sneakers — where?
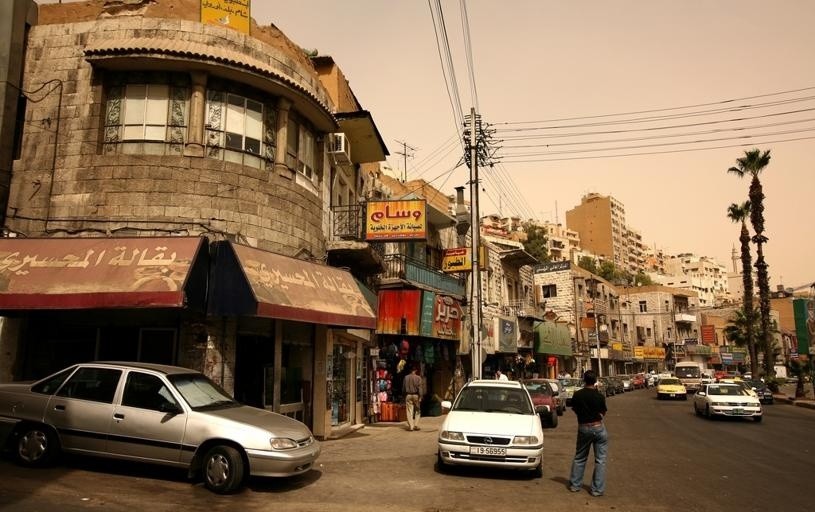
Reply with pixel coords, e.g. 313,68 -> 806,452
571,481 -> 583,491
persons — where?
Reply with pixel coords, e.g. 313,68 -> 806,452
495,370 -> 508,380
569,370 -> 608,496
558,369 -> 572,378
403,366 -> 423,431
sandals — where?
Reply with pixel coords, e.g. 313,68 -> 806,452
413,426 -> 421,430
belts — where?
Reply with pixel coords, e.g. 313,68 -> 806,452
580,421 -> 602,426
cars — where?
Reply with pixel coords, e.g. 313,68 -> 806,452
746,379 -> 775,405
0,360 -> 325,497
565,371 -> 670,403
433,377 -> 550,479
718,376 -> 759,398
692,382 -> 764,422
501,378 -> 559,429
700,369 -> 753,384
533,377 -> 568,417
657,376 -> 687,401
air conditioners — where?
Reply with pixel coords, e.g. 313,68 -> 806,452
327,131 -> 353,167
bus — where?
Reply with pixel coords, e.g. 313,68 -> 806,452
674,361 -> 702,390
